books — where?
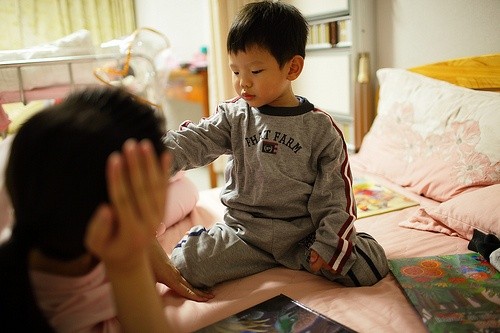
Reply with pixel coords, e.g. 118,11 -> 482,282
385,252 -> 500,333
191,291 -> 361,332
352,181 -> 419,219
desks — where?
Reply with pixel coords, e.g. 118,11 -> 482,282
167,67 -> 217,189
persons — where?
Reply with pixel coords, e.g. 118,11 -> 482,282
0,84 -> 220,332
158,0 -> 389,288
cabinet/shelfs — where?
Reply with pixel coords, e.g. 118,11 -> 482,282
291,0 -> 376,153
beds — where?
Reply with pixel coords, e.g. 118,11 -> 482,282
156,54 -> 500,332
0,53 -> 154,131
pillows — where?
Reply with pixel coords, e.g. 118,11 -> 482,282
352,69 -> 500,204
398,184 -> 500,241
